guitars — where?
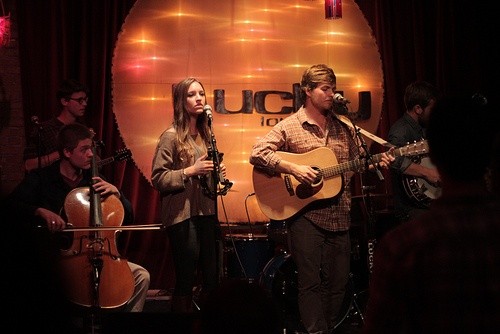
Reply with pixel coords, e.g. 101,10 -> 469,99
252,135 -> 427,220
403,136 -> 444,201
96,146 -> 132,170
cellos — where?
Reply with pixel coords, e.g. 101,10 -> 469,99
62,129 -> 136,309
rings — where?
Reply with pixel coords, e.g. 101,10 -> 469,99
51,220 -> 56,224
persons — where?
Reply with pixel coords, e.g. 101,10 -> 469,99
151,78 -> 227,334
361,84 -> 500,334
389,81 -> 444,222
21,122 -> 150,334
251,65 -> 395,334
24,83 -> 96,186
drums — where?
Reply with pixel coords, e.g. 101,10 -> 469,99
219,222 -> 239,241
223,231 -> 269,279
265,222 -> 287,256
258,251 -> 354,331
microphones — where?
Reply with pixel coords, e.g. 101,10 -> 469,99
31,116 -> 43,131
204,105 -> 213,118
333,93 -> 347,104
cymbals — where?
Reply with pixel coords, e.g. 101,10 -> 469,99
375,208 -> 396,215
200,150 -> 233,197
349,192 -> 390,200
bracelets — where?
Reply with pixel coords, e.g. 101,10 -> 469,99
182,168 -> 190,182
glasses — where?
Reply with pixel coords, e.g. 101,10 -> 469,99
69,96 -> 88,104
